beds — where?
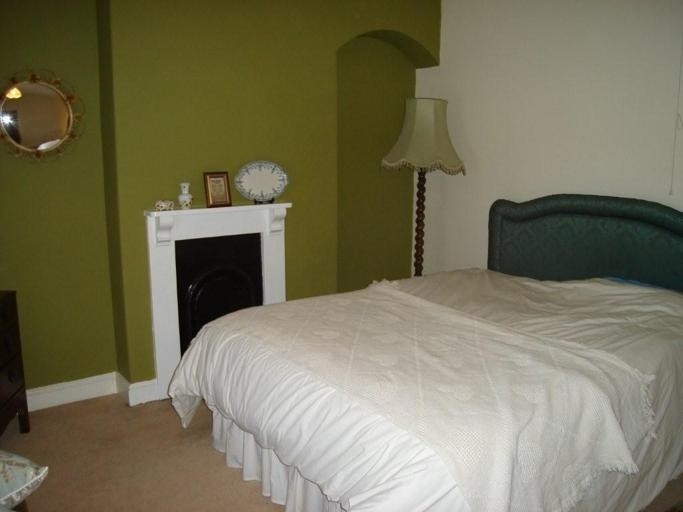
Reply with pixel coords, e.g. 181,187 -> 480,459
167,193 -> 683,512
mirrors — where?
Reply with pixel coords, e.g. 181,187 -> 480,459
0,68 -> 86,164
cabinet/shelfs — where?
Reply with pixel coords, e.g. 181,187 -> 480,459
0,290 -> 30,437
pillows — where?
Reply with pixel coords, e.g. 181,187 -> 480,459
0,449 -> 49,512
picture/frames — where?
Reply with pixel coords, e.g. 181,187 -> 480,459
203,171 -> 232,208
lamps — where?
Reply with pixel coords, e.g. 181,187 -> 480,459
379,98 -> 467,277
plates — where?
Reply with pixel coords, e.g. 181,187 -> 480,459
232,161 -> 288,201
